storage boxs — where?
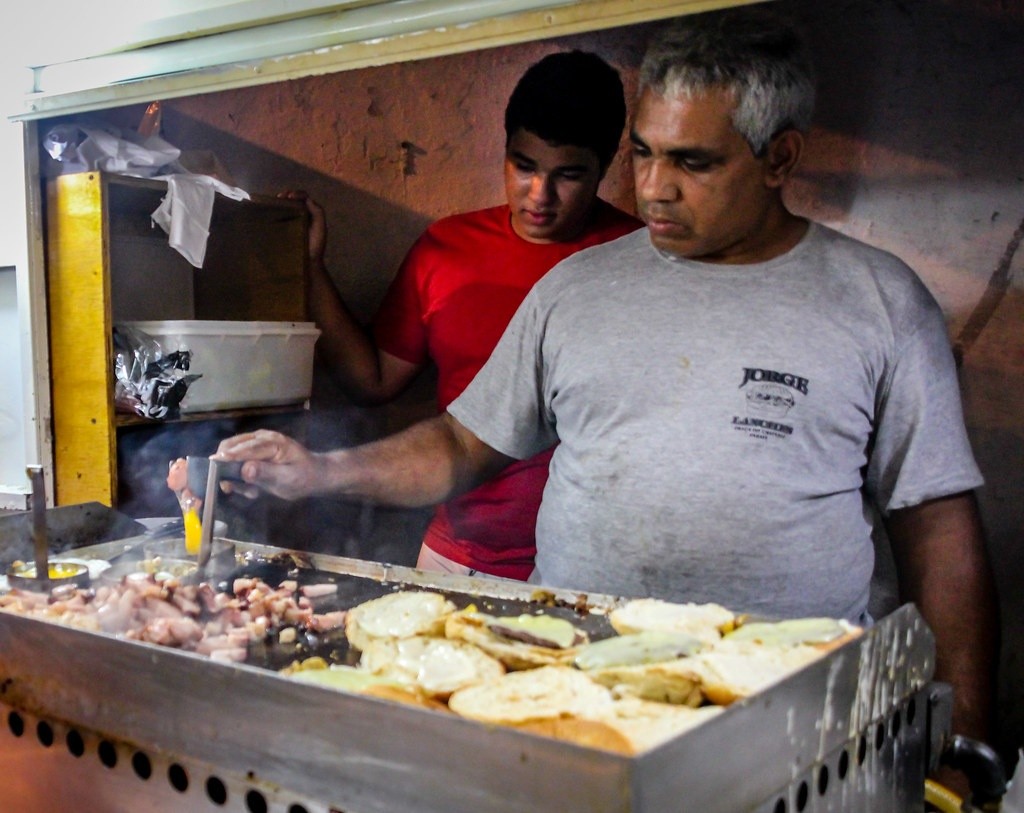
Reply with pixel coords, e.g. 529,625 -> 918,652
119,320 -> 321,414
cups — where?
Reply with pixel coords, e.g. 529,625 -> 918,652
146,537 -> 237,594
99,558 -> 201,590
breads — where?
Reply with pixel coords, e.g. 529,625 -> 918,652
279,590 -> 862,757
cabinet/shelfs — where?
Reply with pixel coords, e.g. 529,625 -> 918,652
44,170 -> 313,511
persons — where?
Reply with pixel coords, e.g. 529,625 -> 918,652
208,32 -> 986,813
277,48 -> 642,587
